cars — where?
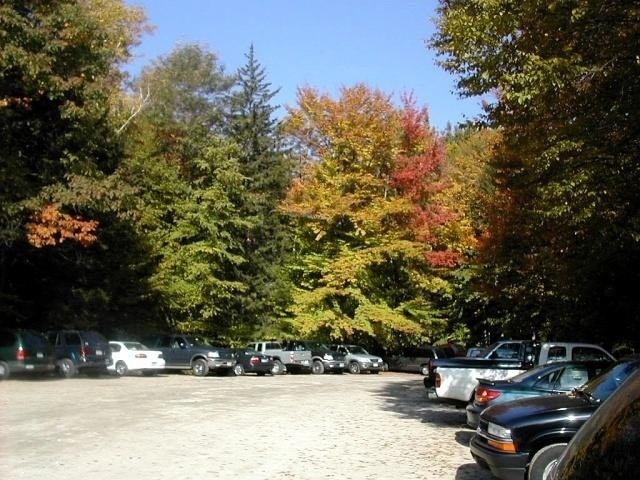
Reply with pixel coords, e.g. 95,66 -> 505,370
216,348 -> 273,376
424,339 -> 640,480
105,340 -> 168,377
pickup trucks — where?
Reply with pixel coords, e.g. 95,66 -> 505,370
247,341 -> 313,374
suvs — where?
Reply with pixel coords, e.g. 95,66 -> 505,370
146,335 -> 238,376
41,330 -> 112,377
1,329 -> 56,380
285,339 -> 344,374
330,344 -> 383,374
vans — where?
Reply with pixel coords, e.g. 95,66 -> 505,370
382,347 -> 444,376
